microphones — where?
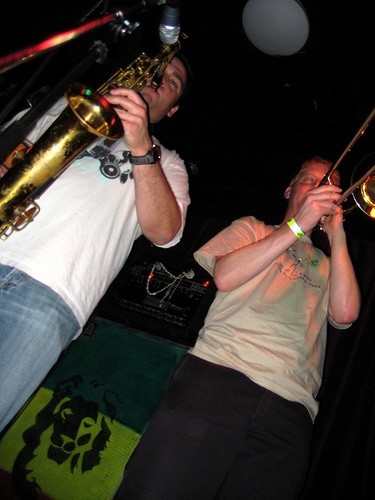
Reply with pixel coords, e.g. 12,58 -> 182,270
159,0 -> 183,44
181,260 -> 195,280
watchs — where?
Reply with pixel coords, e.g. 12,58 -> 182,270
128,145 -> 161,164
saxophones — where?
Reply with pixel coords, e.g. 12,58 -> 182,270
0,31 -> 191,241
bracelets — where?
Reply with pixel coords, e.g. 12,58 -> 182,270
286,218 -> 305,239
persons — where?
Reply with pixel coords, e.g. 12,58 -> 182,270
0,50 -> 190,432
118,158 -> 361,500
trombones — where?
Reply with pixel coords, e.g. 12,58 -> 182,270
316,108 -> 375,219
